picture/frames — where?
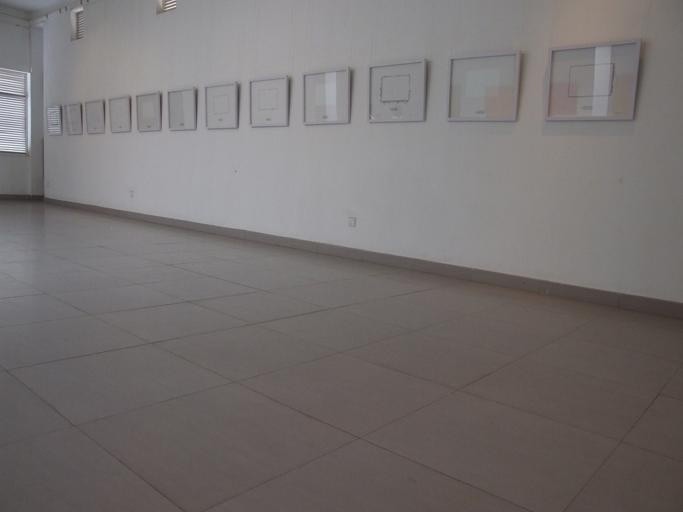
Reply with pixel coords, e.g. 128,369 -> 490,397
134,76 -> 293,133
301,58 -> 431,126
44,95 -> 131,138
445,38 -> 641,124
